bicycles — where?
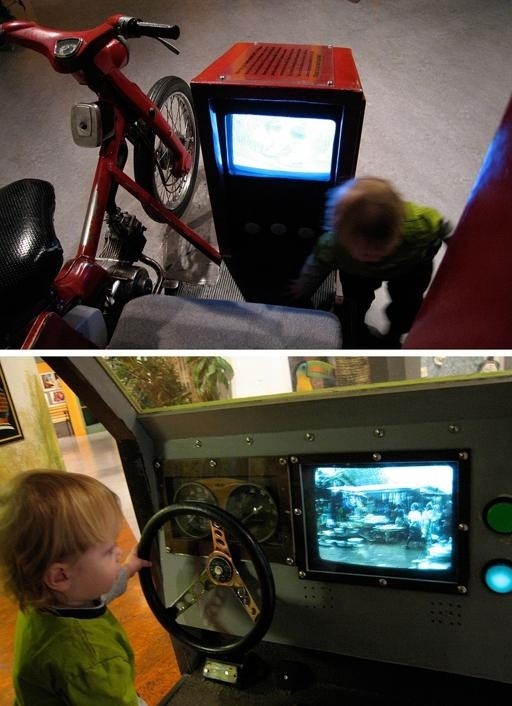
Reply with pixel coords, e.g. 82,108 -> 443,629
1,0 -> 221,349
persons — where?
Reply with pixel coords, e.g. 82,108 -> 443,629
297,174 -> 454,350
0,468 -> 157,706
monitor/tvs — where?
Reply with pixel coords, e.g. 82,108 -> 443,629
302,454 -> 464,588
218,103 -> 338,179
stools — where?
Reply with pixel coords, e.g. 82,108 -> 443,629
20,297 -> 345,351
